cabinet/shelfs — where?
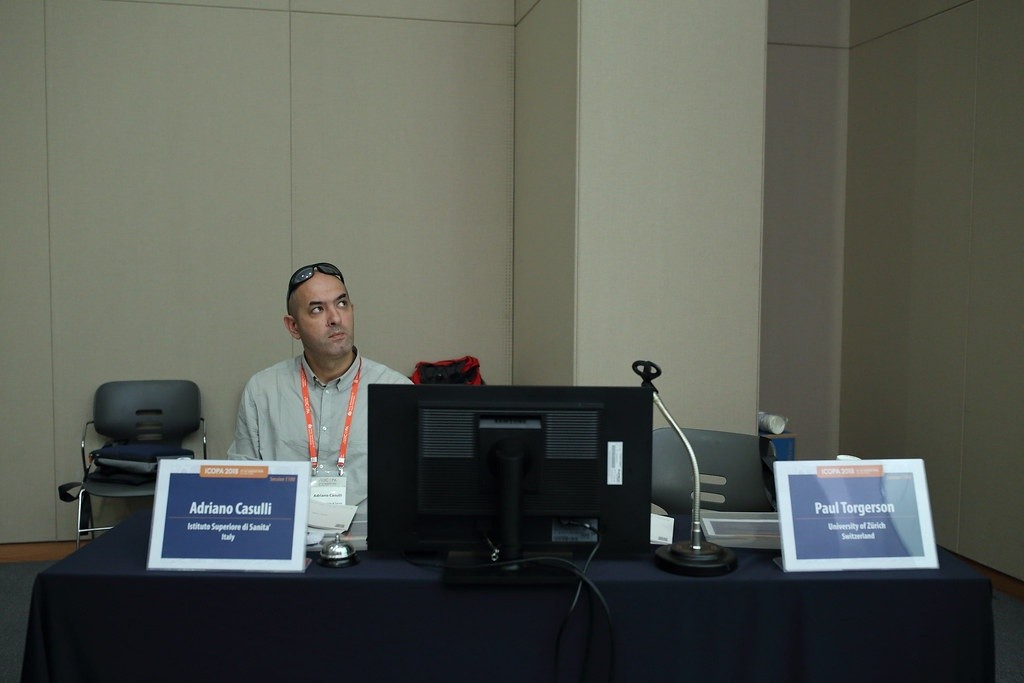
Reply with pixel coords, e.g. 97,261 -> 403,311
760,431 -> 797,499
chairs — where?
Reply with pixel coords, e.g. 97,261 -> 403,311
649,424 -> 768,519
71,378 -> 209,548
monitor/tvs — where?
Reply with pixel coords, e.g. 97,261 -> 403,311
367,383 -> 653,584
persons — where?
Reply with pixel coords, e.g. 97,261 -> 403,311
226,263 -> 415,535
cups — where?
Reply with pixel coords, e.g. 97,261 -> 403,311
836,455 -> 861,459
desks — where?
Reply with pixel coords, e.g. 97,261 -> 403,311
32,509 -> 994,683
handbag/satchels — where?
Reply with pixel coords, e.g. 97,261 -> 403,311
57,443 -> 194,537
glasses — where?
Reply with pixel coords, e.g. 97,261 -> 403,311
287,263 -> 343,297
